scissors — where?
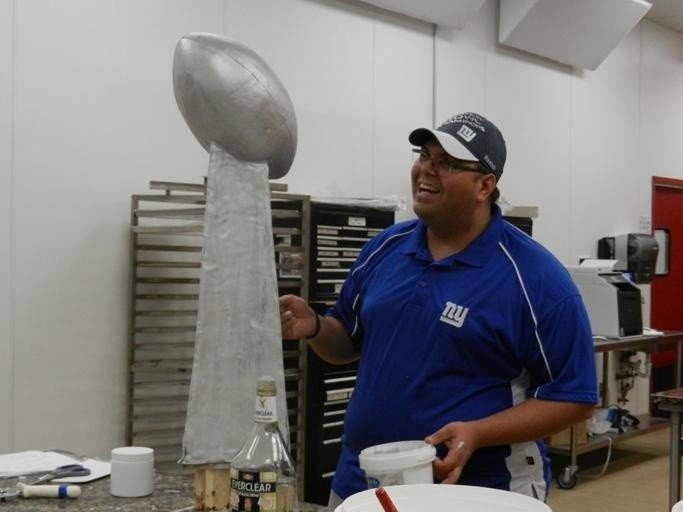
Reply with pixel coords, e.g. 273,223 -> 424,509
31,464 -> 90,485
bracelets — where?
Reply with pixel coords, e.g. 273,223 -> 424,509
308,314 -> 322,340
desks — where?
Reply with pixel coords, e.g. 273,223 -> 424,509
0,459 -> 334,512
651,384 -> 682,510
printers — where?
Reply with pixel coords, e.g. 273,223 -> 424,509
566,267 -> 644,339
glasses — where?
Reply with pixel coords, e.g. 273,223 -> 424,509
411,148 -> 487,175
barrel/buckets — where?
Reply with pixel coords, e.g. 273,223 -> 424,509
358,440 -> 437,490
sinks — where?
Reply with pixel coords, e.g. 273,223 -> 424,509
333,482 -> 555,512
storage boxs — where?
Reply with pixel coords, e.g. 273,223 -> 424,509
547,417 -> 589,447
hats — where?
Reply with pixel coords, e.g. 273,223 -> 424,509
408,112 -> 506,171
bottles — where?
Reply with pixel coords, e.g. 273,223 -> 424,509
225,380 -> 297,511
111,447 -> 154,497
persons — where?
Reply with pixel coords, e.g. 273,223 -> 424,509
274,109 -> 597,511
